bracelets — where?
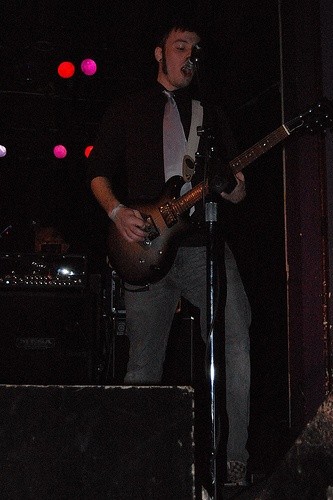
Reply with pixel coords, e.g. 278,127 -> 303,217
109,202 -> 125,221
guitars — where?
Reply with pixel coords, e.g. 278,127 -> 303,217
96,97 -> 333,288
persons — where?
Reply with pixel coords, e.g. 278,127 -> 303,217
86,15 -> 252,500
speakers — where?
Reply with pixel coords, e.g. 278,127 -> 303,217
0,291 -> 203,500
254,390 -> 333,500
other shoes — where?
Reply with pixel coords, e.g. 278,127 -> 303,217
225,460 -> 246,483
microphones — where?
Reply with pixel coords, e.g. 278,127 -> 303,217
186,40 -> 207,70
